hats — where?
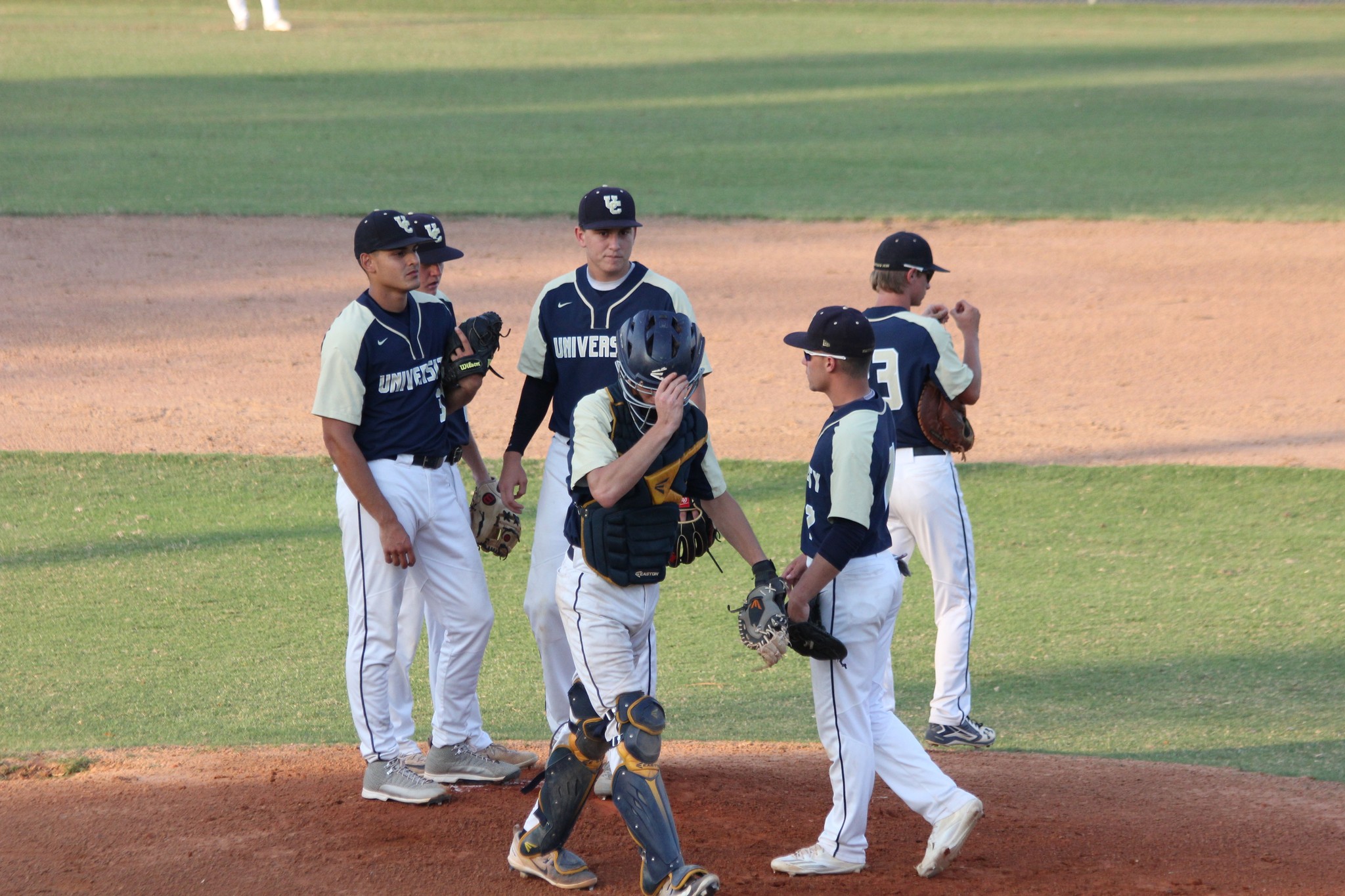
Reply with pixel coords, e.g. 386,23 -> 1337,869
578,186 -> 642,229
873,232 -> 950,273
784,306 -> 875,357
355,209 -> 438,259
409,212 -> 464,263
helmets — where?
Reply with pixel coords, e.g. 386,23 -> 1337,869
615,309 -> 705,386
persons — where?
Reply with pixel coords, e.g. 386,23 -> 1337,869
768,303 -> 985,879
507,309 -> 789,896
853,231 -> 997,751
311,207 -> 521,805
389,211 -> 539,780
497,187 -> 713,797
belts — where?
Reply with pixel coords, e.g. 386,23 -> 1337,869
384,453 -> 444,469
913,446 -> 945,456
567,546 -> 574,560
445,447 -> 464,464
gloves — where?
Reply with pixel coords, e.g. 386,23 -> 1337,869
752,559 -> 786,609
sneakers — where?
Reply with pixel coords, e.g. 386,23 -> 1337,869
657,872 -> 719,896
424,734 -> 522,784
771,844 -> 863,877
361,756 -> 446,806
925,714 -> 997,748
594,761 -> 613,794
399,753 -> 427,776
507,825 -> 598,891
476,743 -> 537,767
917,800 -> 983,877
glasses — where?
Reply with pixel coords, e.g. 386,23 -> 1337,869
904,263 -> 934,283
804,350 -> 847,361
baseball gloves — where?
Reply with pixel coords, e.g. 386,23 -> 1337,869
442,311 -> 504,388
783,596 -> 848,663
917,378 -> 976,453
734,570 -> 788,667
469,476 -> 521,559
667,493 -> 721,569
